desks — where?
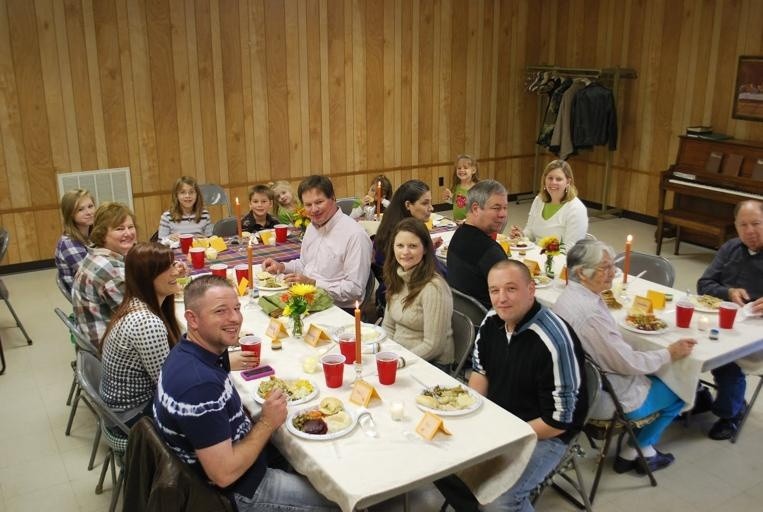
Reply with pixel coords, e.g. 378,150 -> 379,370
673,135 -> 763,252
656,164 -> 763,261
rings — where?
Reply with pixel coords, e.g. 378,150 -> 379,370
243,363 -> 248,369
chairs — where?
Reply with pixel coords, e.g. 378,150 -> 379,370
354,270 -> 591,511
0,229 -> 33,375
54,267 -> 238,512
552,349 -> 657,511
700,374 -> 762,444
614,250 -> 676,288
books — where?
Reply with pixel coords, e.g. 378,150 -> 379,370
705,149 -> 763,180
686,124 -> 735,142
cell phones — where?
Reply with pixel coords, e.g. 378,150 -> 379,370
240,364 -> 275,380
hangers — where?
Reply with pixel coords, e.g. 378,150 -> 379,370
524,72 -> 559,95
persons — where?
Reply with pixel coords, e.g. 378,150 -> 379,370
260,175 -> 373,319
444,180 -> 508,309
508,160 -> 590,256
70,201 -> 139,353
370,177 -> 447,317
156,175 -> 213,246
53,188 -> 96,295
468,258 -> 589,511
97,241 -> 262,440
440,154 -> 482,227
380,218 -> 456,376
551,238 -> 699,474
674,200 -> 762,439
267,179 -> 305,225
347,175 -> 392,219
236,184 -> 281,234
149,274 -> 367,512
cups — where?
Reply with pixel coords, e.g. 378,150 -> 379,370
273,225 -> 288,244
179,232 -> 250,289
376,352 -> 398,387
676,301 -> 694,328
697,316 -> 718,340
323,335 -> 358,387
717,302 -> 739,329
239,335 -> 262,367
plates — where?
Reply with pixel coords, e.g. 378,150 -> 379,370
256,228 -> 290,238
621,310 -> 674,336
255,375 -> 357,441
333,322 -> 388,342
432,214 -> 455,226
255,278 -> 293,290
686,294 -> 728,312
413,381 -> 486,415
433,233 -> 622,289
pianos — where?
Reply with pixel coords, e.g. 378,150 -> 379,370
654,134 -> 763,251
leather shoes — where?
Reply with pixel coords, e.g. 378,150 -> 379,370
635,448 -> 675,475
673,386 -> 714,422
707,398 -> 748,440
612,456 -> 636,473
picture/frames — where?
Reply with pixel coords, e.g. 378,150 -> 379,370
732,56 -> 763,121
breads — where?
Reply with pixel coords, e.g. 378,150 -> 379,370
322,412 -> 351,432
417,396 -> 439,409
320,397 -> 343,415
303,419 -> 327,434
